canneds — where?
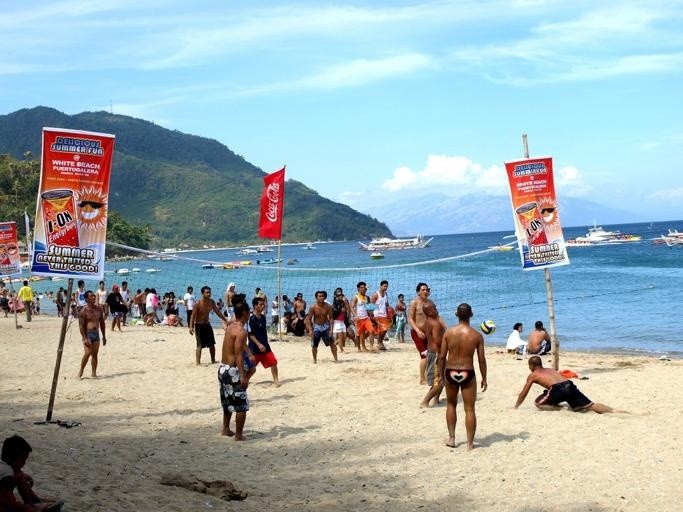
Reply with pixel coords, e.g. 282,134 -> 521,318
0,244 -> 11,265
516,203 -> 548,246
41,190 -> 79,248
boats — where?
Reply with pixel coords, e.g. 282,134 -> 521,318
651,228 -> 683,245
370,252 -> 384,259
358,233 -> 434,250
257,258 -> 283,263
118,267 -> 161,277
235,248 -> 256,256
257,246 -> 274,252
568,220 -> 641,244
151,254 -> 175,261
201,261 -> 253,269
306,244 -> 312,250
1,273 -> 62,285
647,219 -> 653,230
488,246 -> 513,252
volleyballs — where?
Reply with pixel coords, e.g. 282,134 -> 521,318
480,319 -> 496,335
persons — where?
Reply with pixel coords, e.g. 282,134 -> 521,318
420,301 -> 445,408
510,356 -> 648,416
409,283 -> 447,385
528,321 -> 551,355
217,303 -> 250,441
439,303 -> 488,452
507,323 -> 529,355
0,436 -> 64,512
